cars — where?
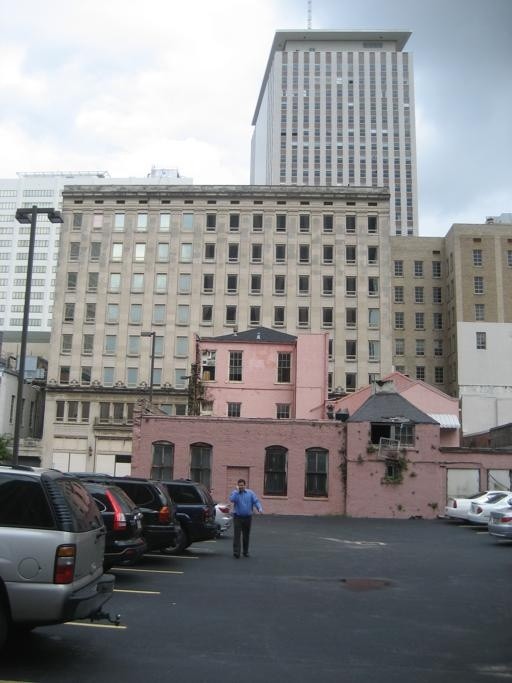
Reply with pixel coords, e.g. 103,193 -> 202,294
211,503 -> 234,537
444,489 -> 511,542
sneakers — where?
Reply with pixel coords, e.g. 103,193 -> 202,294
233,551 -> 249,559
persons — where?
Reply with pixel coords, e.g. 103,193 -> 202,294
230,478 -> 264,559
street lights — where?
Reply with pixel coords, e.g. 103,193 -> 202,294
10,204 -> 67,466
140,328 -> 158,404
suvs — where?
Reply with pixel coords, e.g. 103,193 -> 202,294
2,463 -> 121,648
81,483 -> 146,571
157,478 -> 222,550
69,473 -> 184,553
